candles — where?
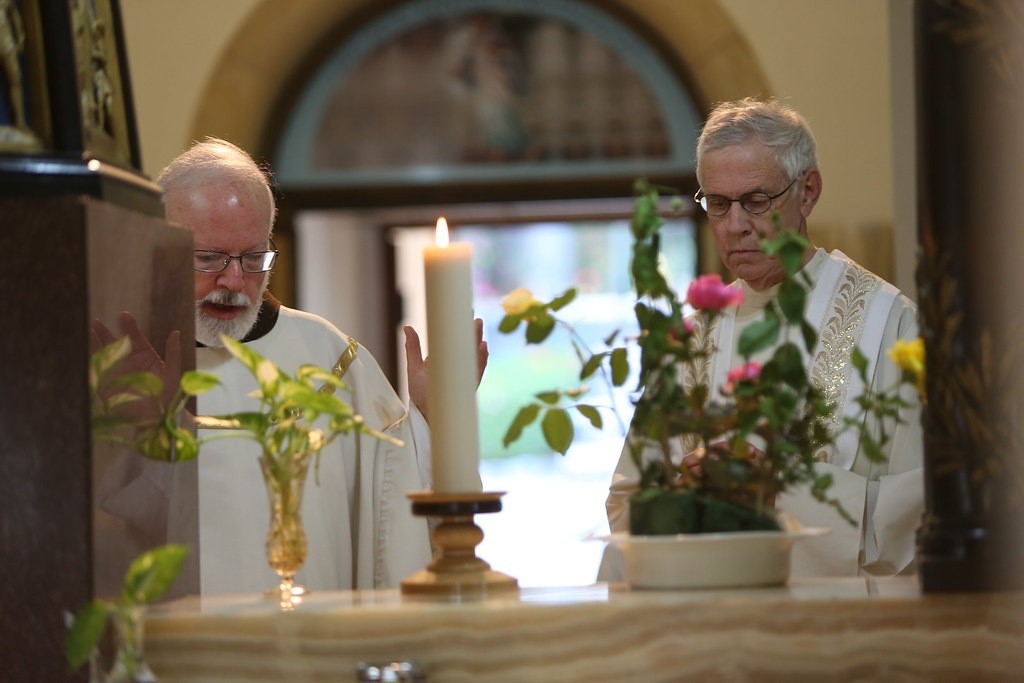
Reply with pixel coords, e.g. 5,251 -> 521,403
423,215 -> 482,492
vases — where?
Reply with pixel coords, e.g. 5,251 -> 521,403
258,453 -> 313,598
598,527 -> 831,590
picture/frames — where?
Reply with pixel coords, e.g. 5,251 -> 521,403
37,0 -> 143,171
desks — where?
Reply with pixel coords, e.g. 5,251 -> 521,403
114,591 -> 1024,683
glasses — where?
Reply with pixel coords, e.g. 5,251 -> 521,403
192,237 -> 280,273
694,173 -> 798,217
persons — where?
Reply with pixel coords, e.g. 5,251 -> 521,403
157,135 -> 489,596
605,97 -> 924,577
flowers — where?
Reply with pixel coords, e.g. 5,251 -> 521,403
502,176 -> 928,531
